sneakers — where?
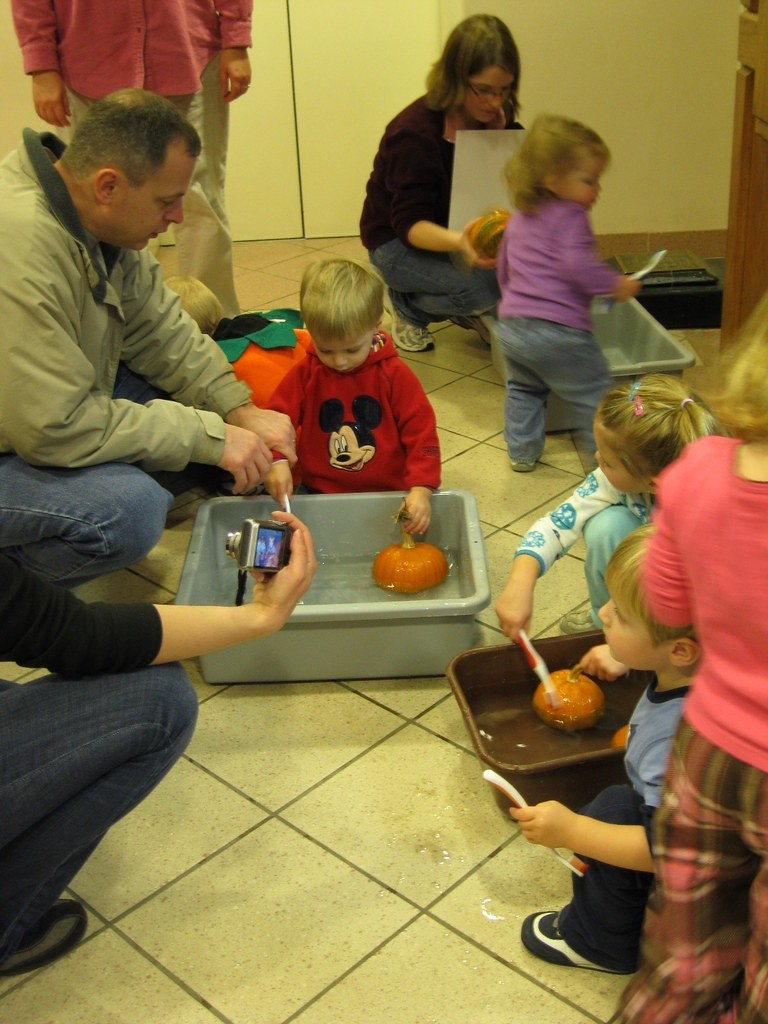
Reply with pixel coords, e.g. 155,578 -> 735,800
451,313 -> 493,345
382,285 -> 435,353
519,910 -> 641,974
511,458 -> 537,471
1,899 -> 87,979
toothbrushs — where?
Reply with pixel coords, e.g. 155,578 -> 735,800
590,251 -> 671,316
479,766 -> 591,879
515,626 -> 565,713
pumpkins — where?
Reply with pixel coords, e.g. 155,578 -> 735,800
371,500 -> 446,594
531,656 -> 605,733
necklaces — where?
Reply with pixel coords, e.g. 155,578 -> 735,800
445,122 -> 482,143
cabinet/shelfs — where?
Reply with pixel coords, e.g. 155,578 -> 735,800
717,0 -> 767,357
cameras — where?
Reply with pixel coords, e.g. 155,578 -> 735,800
224,517 -> 294,575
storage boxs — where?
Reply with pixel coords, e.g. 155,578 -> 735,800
171,485 -> 490,689
614,262 -> 723,336
445,622 -> 659,820
478,287 -> 692,438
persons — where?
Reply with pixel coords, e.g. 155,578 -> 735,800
1,509 -> 321,978
257,258 -> 440,539
360,13 -> 526,352
602,281 -> 765,1024
0,84 -> 299,580
9,0 -> 253,340
490,368 -> 732,639
257,536 -> 282,568
506,525 -> 700,974
496,115 -> 641,477
